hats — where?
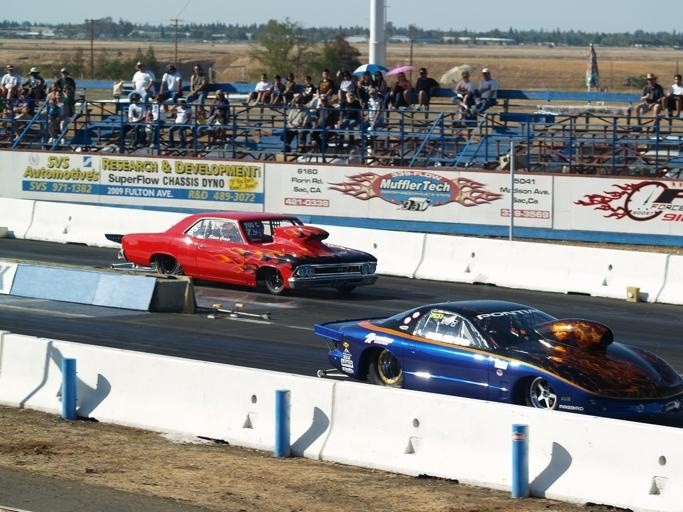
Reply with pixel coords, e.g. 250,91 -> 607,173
645,73 -> 657,81
482,68 -> 490,74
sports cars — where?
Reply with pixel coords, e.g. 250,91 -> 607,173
97,210 -> 383,295
301,298 -> 682,423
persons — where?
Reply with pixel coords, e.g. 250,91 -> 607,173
662,74 -> 683,117
633,73 -> 664,132
450,71 -> 476,127
472,68 -> 498,116
0,64 -> 76,144
109,61 -> 230,150
241,68 -> 441,152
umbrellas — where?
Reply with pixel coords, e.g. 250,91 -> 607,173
354,64 -> 389,77
384,65 -> 413,77
440,64 -> 473,85
